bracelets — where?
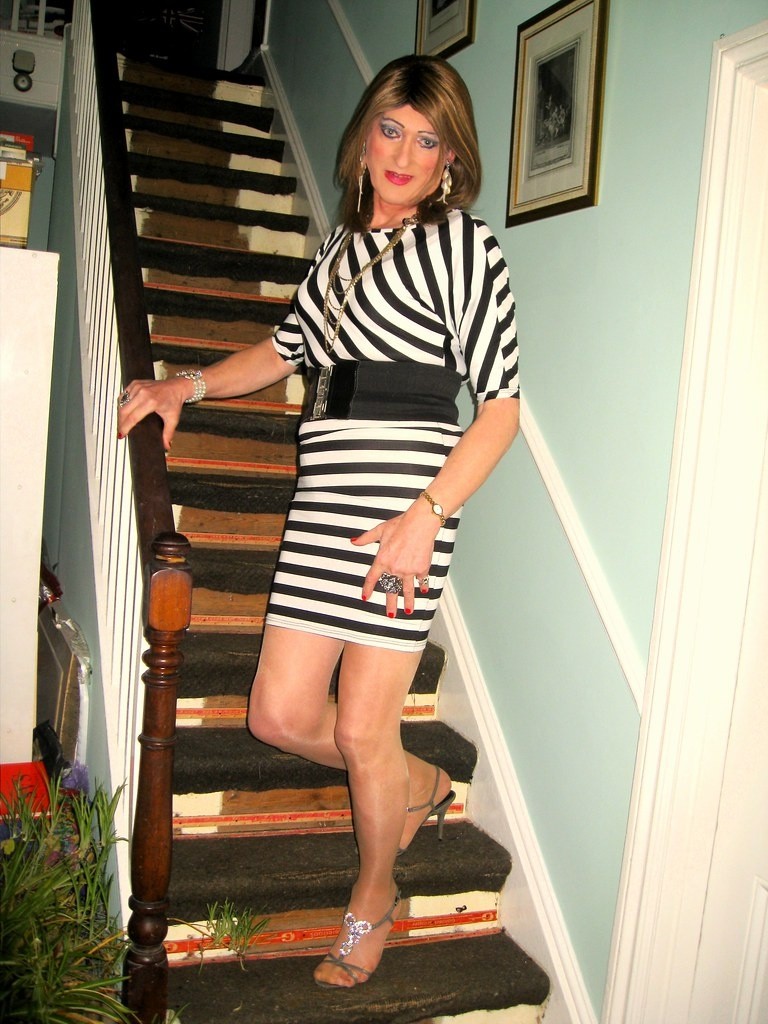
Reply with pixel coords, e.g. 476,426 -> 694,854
176,368 -> 206,403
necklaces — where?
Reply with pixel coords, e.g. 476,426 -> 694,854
323,212 -> 418,353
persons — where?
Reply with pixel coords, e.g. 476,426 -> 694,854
117,55 -> 522,989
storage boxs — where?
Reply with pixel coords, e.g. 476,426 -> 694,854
0,131 -> 36,248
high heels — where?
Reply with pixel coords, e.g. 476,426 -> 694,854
315,884 -> 402,990
355,763 -> 456,860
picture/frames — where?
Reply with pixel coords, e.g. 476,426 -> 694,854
506,0 -> 610,229
414,0 -> 478,59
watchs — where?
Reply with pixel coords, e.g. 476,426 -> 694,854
419,492 -> 446,526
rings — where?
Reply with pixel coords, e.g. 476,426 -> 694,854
378,572 -> 403,593
119,391 -> 130,408
418,577 -> 429,584
